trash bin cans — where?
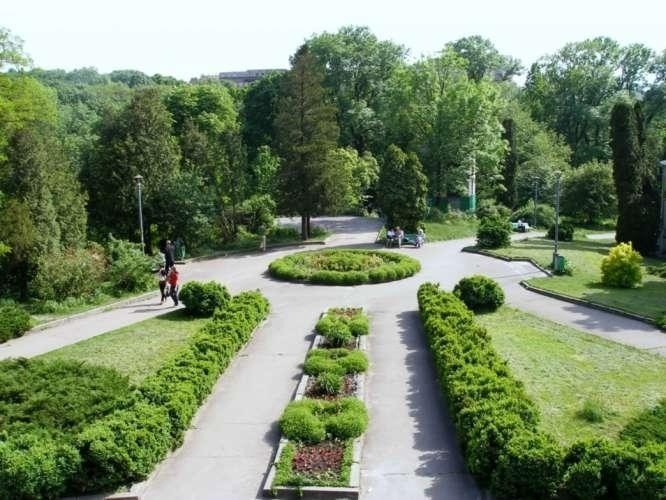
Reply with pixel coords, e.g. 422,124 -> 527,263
555,255 -> 564,274
175,244 -> 185,260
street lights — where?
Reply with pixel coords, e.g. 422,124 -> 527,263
553,170 -> 561,273
132,173 -> 146,257
533,175 -> 539,231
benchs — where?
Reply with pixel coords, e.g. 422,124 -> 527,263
384,233 -> 417,248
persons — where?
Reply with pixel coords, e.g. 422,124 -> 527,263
386,226 -> 403,248
165,240 -> 174,273
416,229 -> 425,247
159,265 -> 179,306
518,220 -> 525,232
